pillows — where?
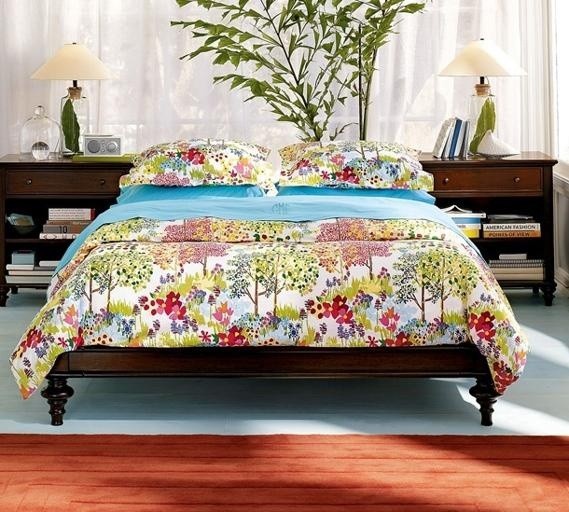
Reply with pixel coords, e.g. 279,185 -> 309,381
282,138 -> 434,191
119,139 -> 281,190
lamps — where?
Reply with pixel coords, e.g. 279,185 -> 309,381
437,41 -> 528,157
28,42 -> 108,156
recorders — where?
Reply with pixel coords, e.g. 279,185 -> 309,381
85,138 -> 121,155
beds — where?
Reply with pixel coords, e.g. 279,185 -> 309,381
9,137 -> 530,427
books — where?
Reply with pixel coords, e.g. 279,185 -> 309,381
35,266 -> 57,270
49,207 -> 96,221
483,224 -> 541,232
40,232 -> 82,240
488,268 -> 546,274
490,259 -> 543,264
448,213 -> 488,219
43,224 -> 87,233
452,219 -> 480,223
9,270 -> 56,277
5,275 -> 55,284
492,274 -> 546,280
6,264 -> 34,270
487,264 -> 545,268
39,260 -> 60,267
483,231 -> 542,238
499,253 -> 527,259
439,204 -> 472,212
488,213 -> 537,223
461,231 -> 479,238
454,224 -> 481,230
46,221 -> 93,225
432,116 -> 470,158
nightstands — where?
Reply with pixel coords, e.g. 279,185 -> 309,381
0,150 -> 139,308
414,150 -> 557,308
